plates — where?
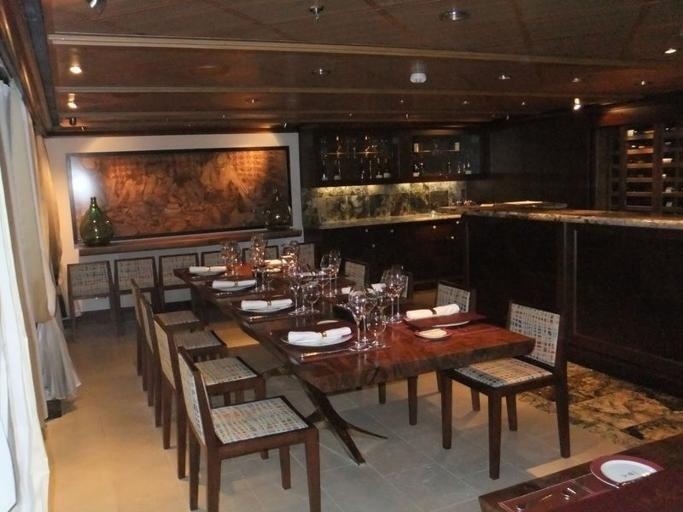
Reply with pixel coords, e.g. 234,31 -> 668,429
237,298 -> 294,314
281,324 -> 357,348
208,280 -> 256,292
404,312 -> 471,328
589,454 -> 664,490
415,328 -> 458,340
189,269 -> 226,277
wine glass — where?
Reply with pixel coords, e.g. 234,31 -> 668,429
348,270 -> 407,352
221,231 -> 341,316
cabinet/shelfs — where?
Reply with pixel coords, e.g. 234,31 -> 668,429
594,121 -> 682,218
322,219 -> 463,292
298,121 -> 490,189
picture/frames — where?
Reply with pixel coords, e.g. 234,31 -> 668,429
63,146 -> 291,247
